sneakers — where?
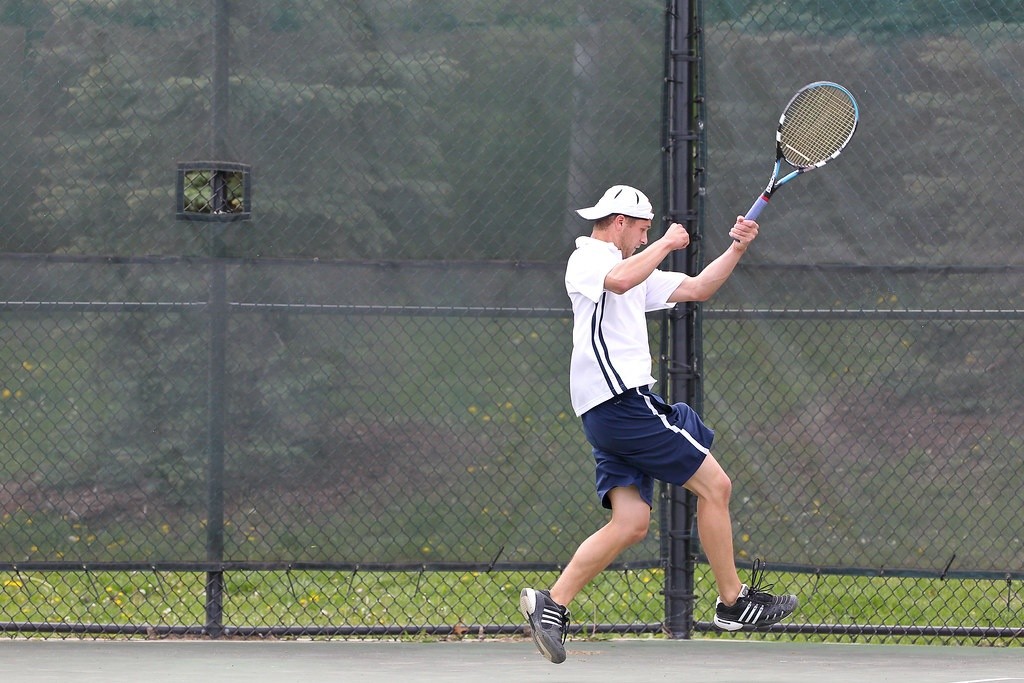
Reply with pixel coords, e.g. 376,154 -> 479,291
713,559 -> 797,632
520,588 -> 570,665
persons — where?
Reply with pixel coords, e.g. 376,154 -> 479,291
517,183 -> 799,665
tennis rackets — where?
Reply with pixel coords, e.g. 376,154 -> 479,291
732,80 -> 859,243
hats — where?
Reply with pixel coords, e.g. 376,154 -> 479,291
575,185 -> 654,220
